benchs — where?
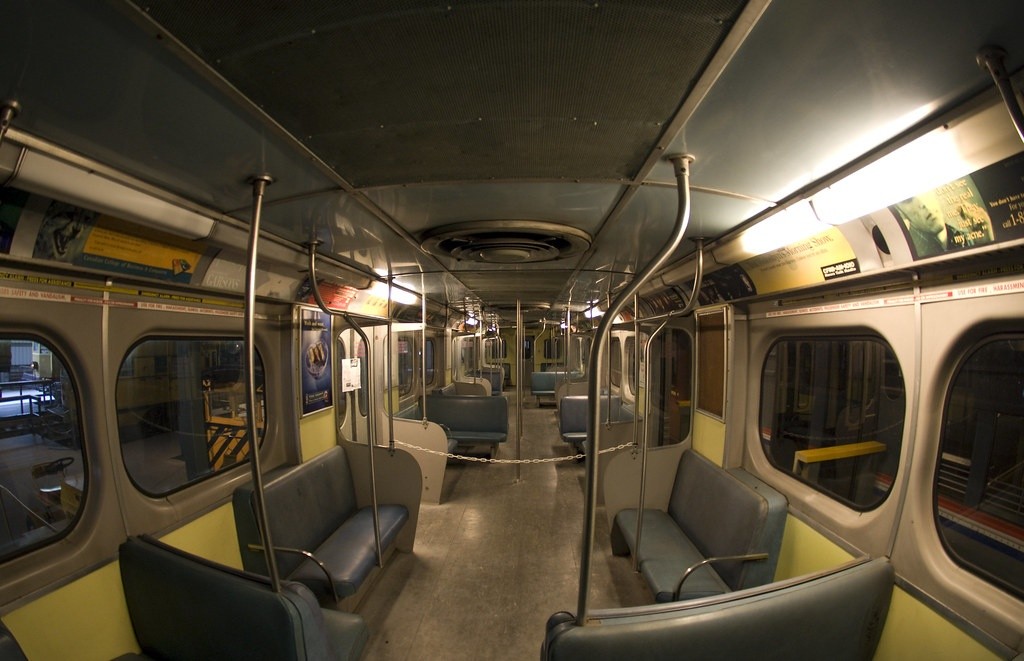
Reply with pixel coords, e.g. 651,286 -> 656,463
117,533 -> 371,661
617,449 -> 785,603
561,396 -> 642,458
540,555 -> 896,661
234,444 -> 410,603
396,363 -> 513,458
532,371 -> 585,407
